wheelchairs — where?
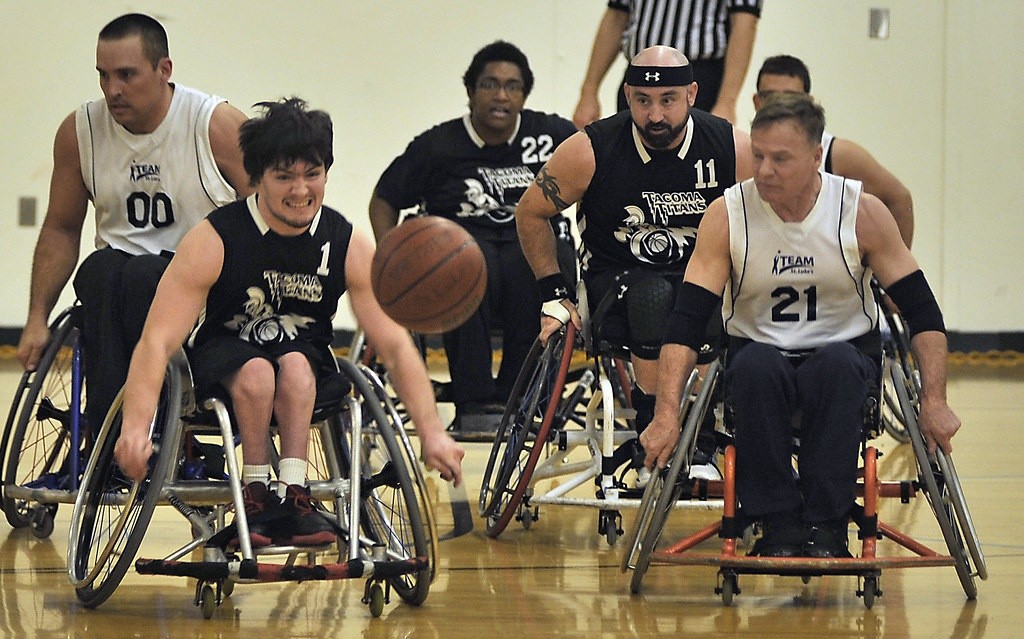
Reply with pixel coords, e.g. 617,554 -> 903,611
0,306 -> 347,568
350,300 -> 988,613
64,354 -> 439,620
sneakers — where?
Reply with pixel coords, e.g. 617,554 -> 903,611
227,481 -> 273,547
271,479 -> 335,545
636,462 -> 655,489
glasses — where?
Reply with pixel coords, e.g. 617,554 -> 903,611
474,79 -> 525,94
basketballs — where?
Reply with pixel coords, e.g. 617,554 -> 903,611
371,217 -> 489,333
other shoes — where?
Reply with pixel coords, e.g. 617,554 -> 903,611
753,522 -> 801,557
804,521 -> 848,559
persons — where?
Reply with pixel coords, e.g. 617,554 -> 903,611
18,12 -> 256,488
574,0 -> 764,128
753,55 -> 914,315
639,91 -> 962,558
113,97 -> 465,546
368,39 -> 579,437
514,44 -> 754,489
